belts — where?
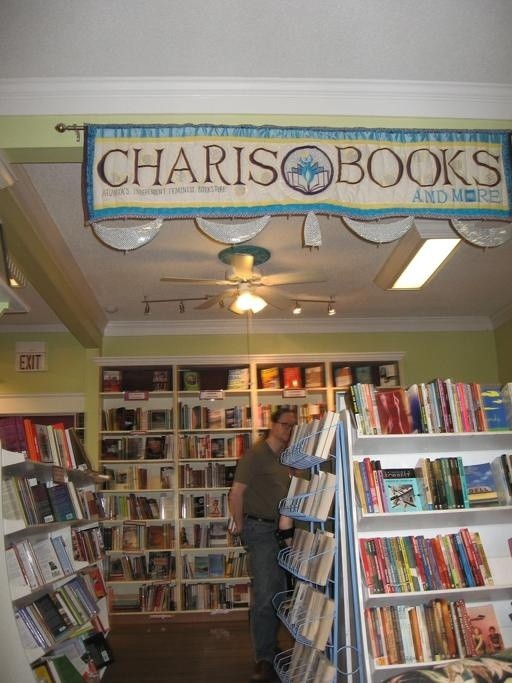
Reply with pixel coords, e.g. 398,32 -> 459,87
245,514 -> 275,523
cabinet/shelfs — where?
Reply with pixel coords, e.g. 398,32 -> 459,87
338,410 -> 512,682
0,459 -> 113,683
96,350 -> 405,633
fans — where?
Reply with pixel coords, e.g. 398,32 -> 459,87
160,251 -> 326,312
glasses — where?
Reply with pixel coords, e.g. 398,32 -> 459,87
277,422 -> 294,429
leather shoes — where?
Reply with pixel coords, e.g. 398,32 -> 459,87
251,660 -> 273,683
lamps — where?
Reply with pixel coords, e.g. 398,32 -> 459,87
373,219 -> 466,293
139,294 -> 336,319
230,283 -> 268,316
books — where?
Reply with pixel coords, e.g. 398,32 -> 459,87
101,364 -> 399,615
286,378 -> 512,683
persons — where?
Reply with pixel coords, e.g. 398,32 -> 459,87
228,409 -> 300,681
0,417 -> 113,683
